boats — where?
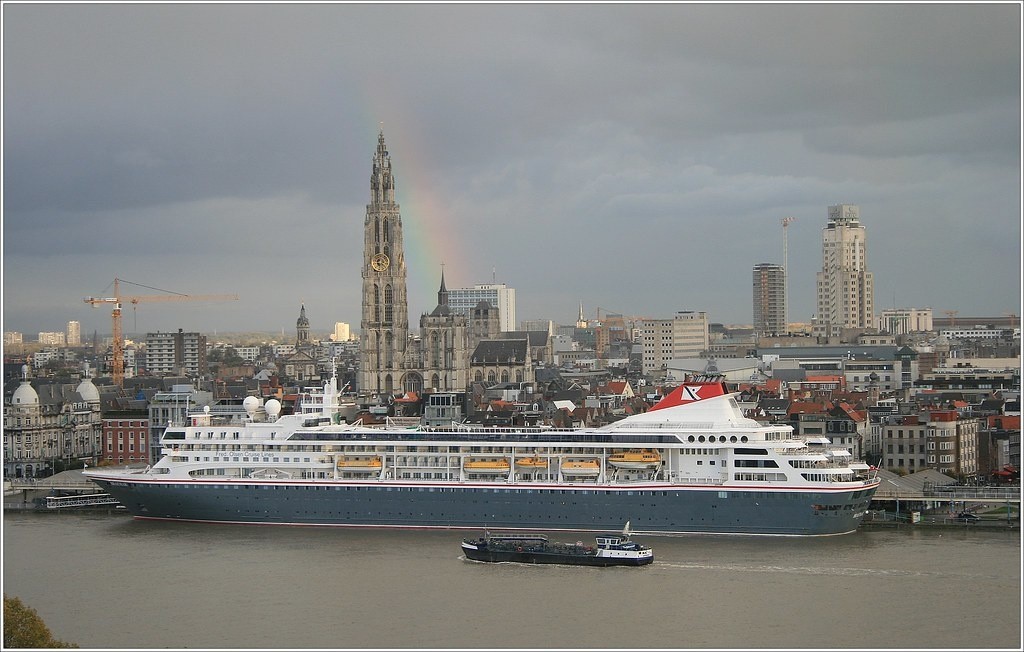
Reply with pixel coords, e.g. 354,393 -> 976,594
459,529 -> 655,567
81,395 -> 881,539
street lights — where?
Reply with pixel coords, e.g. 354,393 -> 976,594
48,439 -> 54,476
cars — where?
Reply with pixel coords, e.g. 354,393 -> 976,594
958,513 -> 982,521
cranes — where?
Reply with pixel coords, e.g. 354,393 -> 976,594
781,218 -> 799,336
82,277 -> 241,388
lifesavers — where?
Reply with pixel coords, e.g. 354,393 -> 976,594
518,547 -> 522,552
